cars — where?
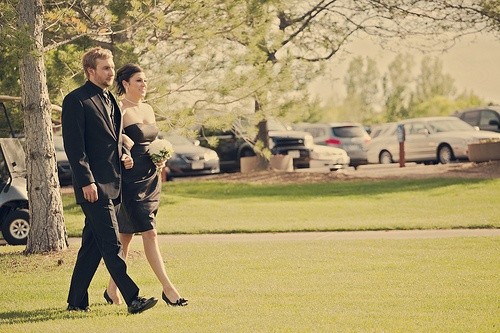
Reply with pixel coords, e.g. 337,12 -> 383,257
199,120 -> 372,174
367,113 -> 499,164
157,128 -> 222,184
459,107 -> 499,134
0,95 -> 70,246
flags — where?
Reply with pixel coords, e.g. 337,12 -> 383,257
254,96 -> 261,112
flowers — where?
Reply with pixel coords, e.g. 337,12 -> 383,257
146,139 -> 174,172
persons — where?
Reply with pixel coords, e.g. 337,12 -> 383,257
61,43 -> 158,314
103,64 -> 190,308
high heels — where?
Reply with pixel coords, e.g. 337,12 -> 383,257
104,289 -> 113,304
162,291 -> 188,307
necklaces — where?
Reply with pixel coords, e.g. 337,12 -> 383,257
122,96 -> 144,105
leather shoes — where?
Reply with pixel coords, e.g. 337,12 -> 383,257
67,304 -> 91,312
128,296 -> 158,314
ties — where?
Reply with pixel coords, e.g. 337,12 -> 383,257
103,93 -> 112,116
